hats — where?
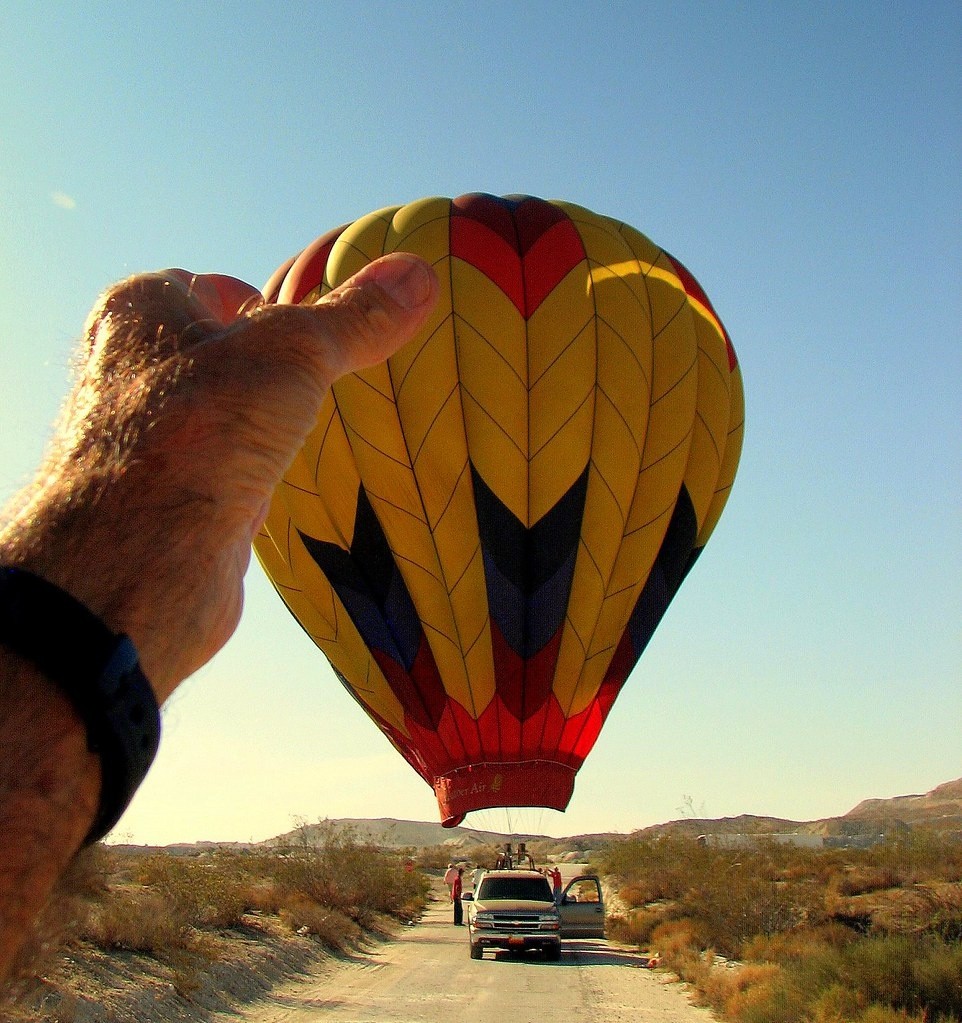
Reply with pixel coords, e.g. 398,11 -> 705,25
458,868 -> 464,873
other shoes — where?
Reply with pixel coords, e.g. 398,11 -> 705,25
456,923 -> 465,928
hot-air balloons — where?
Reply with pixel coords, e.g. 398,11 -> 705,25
253,192 -> 744,871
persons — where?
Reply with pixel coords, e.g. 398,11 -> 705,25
0,253 -> 442,995
470,865 -> 481,889
452,868 -> 465,926
444,864 -> 456,904
539,867 -> 562,899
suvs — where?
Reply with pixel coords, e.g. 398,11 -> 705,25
460,872 -> 606,962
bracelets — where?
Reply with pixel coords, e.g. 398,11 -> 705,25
0,565 -> 160,848
